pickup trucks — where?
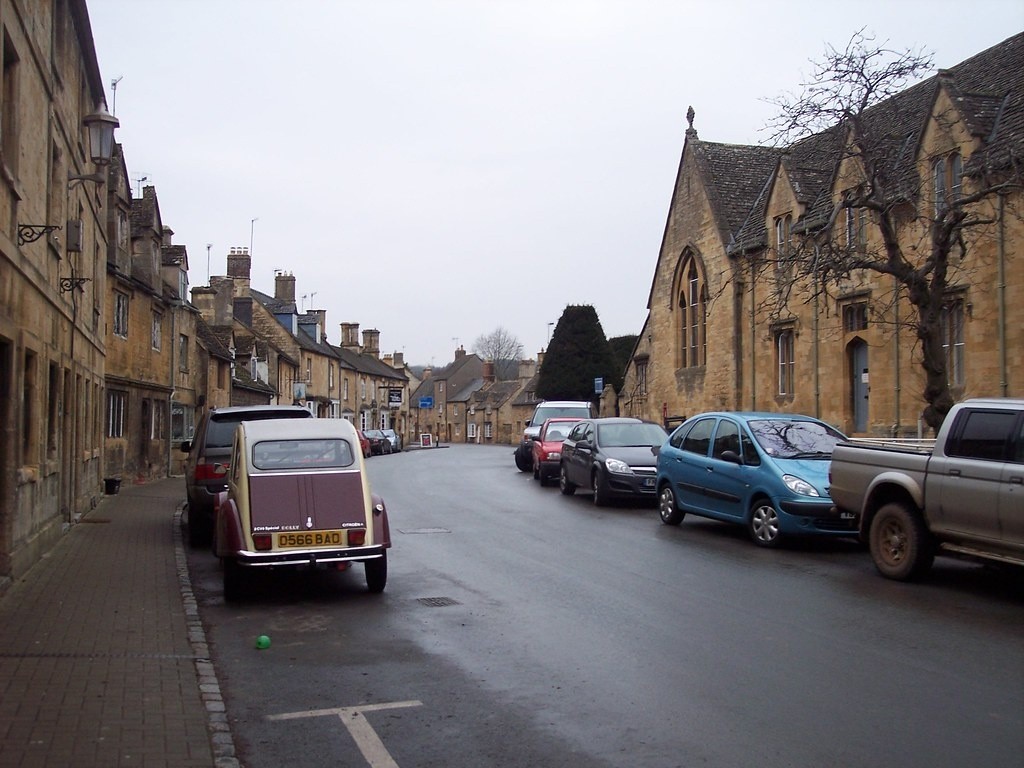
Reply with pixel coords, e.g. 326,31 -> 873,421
829,398 -> 1024,583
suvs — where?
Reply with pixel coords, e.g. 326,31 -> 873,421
180,402 -> 317,548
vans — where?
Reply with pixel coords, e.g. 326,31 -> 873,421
514,401 -> 599,473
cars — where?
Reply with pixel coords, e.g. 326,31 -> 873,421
655,412 -> 861,549
213,417 -> 392,607
532,417 -> 587,488
357,430 -> 372,459
559,417 -> 671,506
362,429 -> 393,456
381,429 -> 402,453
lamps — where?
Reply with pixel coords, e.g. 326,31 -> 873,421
68,97 -> 121,191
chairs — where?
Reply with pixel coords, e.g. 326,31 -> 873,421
549,430 -> 562,441
